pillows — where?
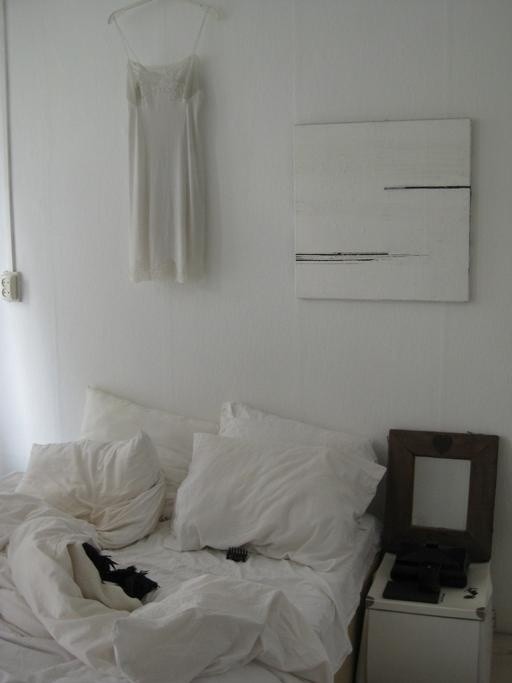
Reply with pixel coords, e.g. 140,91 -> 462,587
78,385 -> 220,515
173,433 -> 387,572
218,401 -> 378,464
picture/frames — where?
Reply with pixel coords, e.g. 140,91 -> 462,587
383,429 -> 501,563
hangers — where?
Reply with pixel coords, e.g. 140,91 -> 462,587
106,1 -> 222,25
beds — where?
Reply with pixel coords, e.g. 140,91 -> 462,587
2,479 -> 383,683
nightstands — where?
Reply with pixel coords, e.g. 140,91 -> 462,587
356,555 -> 496,683
